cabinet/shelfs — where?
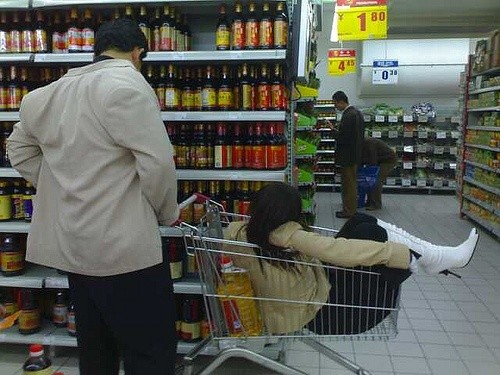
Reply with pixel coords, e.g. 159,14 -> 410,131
0,0 -> 500,362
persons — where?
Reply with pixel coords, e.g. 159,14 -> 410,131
362,136 -> 397,209
7,19 -> 181,375
327,91 -> 364,217
223,183 -> 480,335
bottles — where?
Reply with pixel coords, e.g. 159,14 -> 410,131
22,344 -> 53,375
0,1 -> 288,344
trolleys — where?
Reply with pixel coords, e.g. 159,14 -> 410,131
167,191 -> 402,375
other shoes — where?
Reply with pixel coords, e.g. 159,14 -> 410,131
366,207 -> 375,210
336,211 -> 350,218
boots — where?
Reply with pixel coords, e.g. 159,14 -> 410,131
377,220 -> 476,250
385,229 -> 479,278
